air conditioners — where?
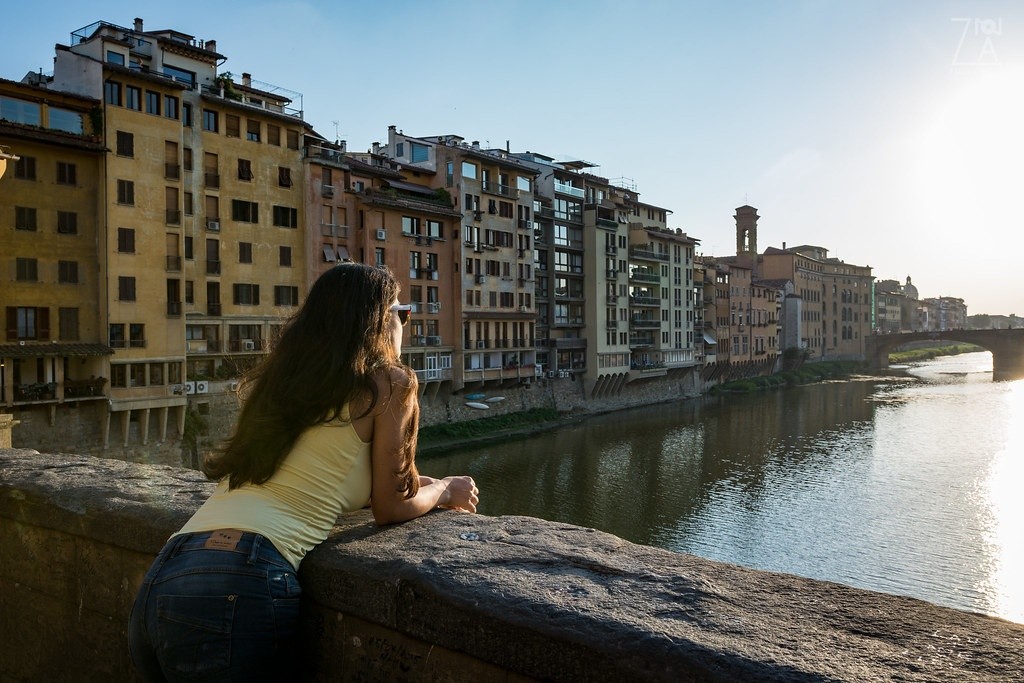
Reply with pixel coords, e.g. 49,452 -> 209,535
197,381 -> 209,393
245,342 -> 255,350
565,372 -> 569,377
560,372 -> 565,377
549,371 -> 554,377
208,221 -> 219,230
521,306 -> 527,311
187,381 -> 195,394
478,341 -> 485,349
427,303 -> 438,313
231,383 -> 240,391
187,342 -> 190,352
436,336 -> 441,345
480,277 -> 485,283
527,221 -> 532,228
376,230 -> 385,240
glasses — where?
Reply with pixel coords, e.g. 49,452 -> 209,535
388,303 -> 412,326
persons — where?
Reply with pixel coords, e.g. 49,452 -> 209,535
126,263 -> 479,683
